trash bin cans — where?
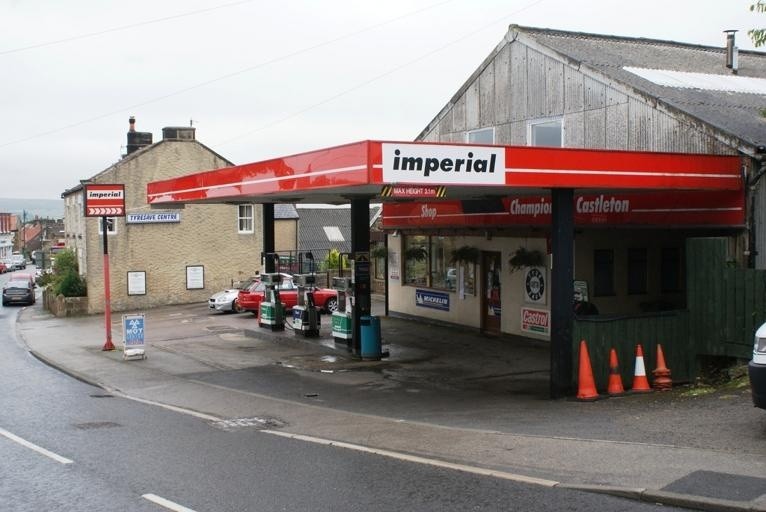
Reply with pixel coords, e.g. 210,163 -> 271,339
360,316 -> 381,360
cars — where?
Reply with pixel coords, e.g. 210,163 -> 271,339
2,280 -> 36,306
10,273 -> 35,286
208,287 -> 249,314
749,320 -> 766,406
238,272 -> 341,314
0,255 -> 26,274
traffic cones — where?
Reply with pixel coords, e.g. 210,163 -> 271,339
575,339 -> 598,399
632,344 -> 652,391
606,348 -> 625,395
652,343 -> 674,388
